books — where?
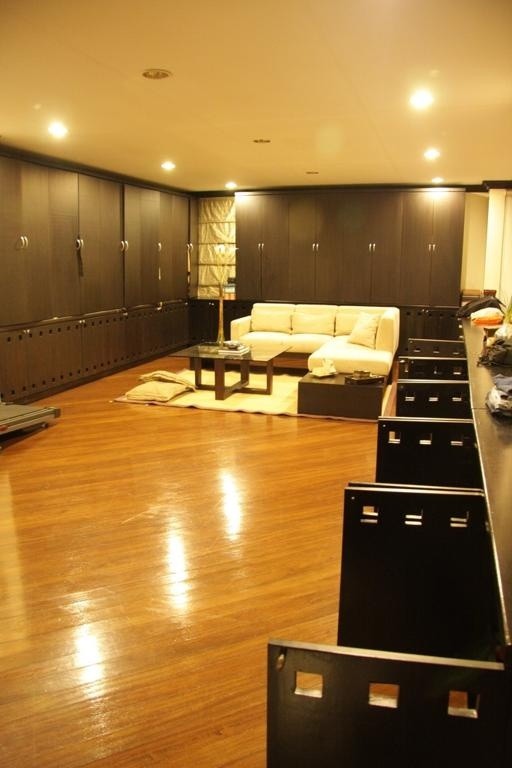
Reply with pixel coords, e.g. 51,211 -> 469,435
197,342 -> 250,357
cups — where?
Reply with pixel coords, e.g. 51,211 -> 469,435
352,368 -> 371,380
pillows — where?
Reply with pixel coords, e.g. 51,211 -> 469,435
350,312 -> 381,349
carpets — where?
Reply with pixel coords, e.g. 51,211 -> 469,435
116,369 -> 393,423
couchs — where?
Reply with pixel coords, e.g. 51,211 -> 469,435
230,301 -> 400,376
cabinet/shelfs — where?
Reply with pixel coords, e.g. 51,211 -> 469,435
0,152 -> 190,326
234,185 -> 466,308
